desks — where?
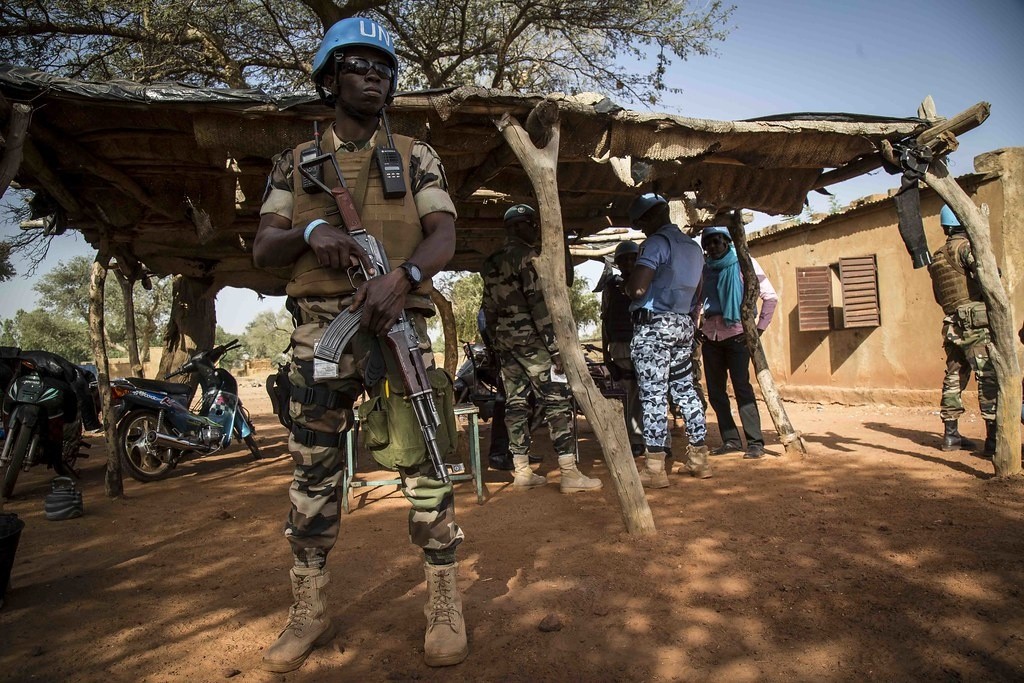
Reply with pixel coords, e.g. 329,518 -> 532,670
340,402 -> 485,513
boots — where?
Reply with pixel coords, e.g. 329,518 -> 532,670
638,450 -> 670,488
557,454 -> 604,494
984,419 -> 997,456
261,567 -> 335,673
423,561 -> 468,666
512,454 -> 547,492
942,420 -> 977,451
678,444 -> 713,478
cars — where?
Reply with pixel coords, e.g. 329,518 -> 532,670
74,363 -> 100,386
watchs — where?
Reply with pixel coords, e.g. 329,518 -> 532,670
400,262 -> 423,289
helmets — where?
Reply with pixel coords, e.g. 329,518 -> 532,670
504,204 -> 536,221
941,204 -> 960,226
614,240 -> 639,265
629,193 -> 670,230
310,18 -> 398,110
700,226 -> 733,250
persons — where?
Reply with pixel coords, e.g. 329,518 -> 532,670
694,226 -> 778,459
928,205 -> 1003,457
625,192 -> 713,488
602,240 -> 672,460
477,203 -> 604,494
252,18 -> 467,673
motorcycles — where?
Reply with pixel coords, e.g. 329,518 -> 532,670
454,339 -> 497,422
88,339 -> 260,483
0,345 -> 108,511
546,334 -> 605,377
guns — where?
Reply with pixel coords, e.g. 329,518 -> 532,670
295,151 -> 457,487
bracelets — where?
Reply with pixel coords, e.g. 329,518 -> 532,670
304,219 -> 329,244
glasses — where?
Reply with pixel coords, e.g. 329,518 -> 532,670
705,237 -> 728,246
509,220 -> 538,230
347,57 -> 394,79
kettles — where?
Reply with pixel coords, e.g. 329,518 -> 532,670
46,477 -> 83,520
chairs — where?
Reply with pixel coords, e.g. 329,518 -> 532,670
572,362 -> 629,463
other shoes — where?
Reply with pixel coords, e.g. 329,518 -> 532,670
631,444 -> 645,457
665,447 -> 673,459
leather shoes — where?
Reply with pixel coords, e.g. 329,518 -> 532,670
708,445 -> 740,454
743,445 -> 765,459
528,454 -> 544,461
489,453 -> 515,469
